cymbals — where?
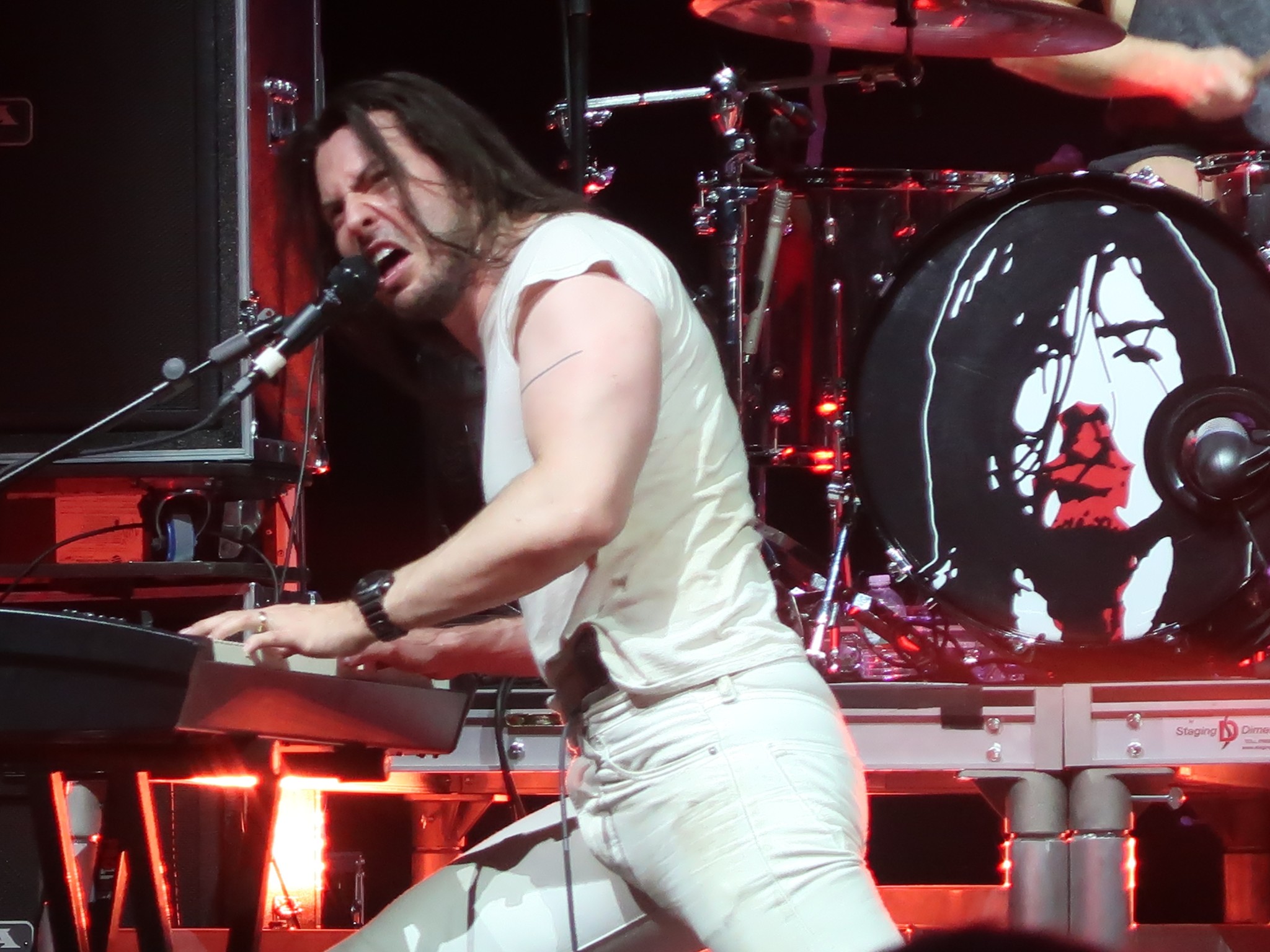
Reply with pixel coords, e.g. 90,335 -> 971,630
687,0 -> 1129,59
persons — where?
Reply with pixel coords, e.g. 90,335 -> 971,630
177,71 -> 911,951
990,2 -> 1270,150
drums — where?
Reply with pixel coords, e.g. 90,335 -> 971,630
723,166 -> 1025,477
1195,151 -> 1269,260
840,164 -> 1270,651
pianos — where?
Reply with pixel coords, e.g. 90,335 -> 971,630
2,602 -> 484,952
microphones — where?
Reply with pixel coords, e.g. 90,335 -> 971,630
218,254 -> 379,410
744,185 -> 795,369
1195,417 -> 1251,500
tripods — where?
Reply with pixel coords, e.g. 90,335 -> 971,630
693,66 -> 978,685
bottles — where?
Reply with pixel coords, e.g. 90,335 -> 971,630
858,575 -> 914,682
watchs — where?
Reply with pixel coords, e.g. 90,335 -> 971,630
351,569 -> 409,643
